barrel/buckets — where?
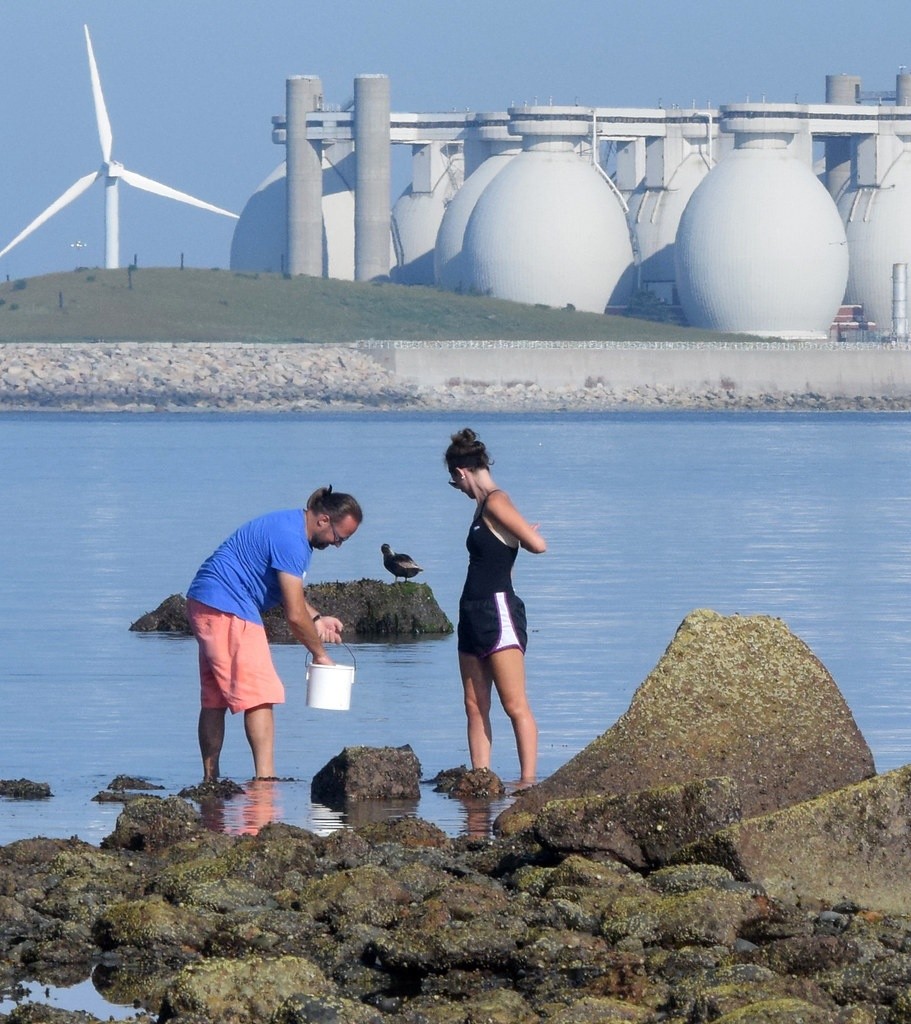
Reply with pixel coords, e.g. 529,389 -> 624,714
305,636 -> 357,710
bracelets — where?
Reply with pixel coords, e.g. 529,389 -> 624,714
313,613 -> 321,622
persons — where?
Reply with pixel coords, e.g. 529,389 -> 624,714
446,429 -> 547,780
187,485 -> 363,780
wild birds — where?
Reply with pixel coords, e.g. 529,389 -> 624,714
380,543 -> 422,583
